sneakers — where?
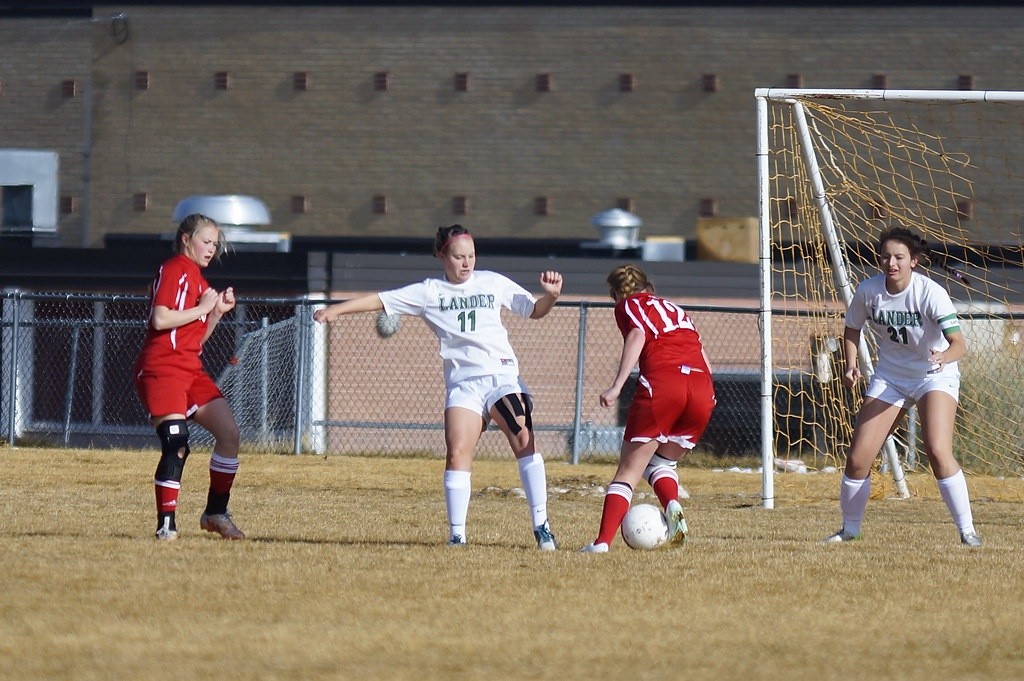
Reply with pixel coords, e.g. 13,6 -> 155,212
155,527 -> 177,540
533,520 -> 556,552
448,534 -> 465,545
580,538 -> 609,554
825,529 -> 855,543
663,500 -> 689,544
961,532 -> 983,546
199,510 -> 246,540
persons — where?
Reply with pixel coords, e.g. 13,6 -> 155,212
820,226 -> 983,551
313,224 -> 562,551
139,215 -> 249,542
577,264 -> 718,554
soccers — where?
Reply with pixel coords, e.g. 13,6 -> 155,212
621,503 -> 671,551
